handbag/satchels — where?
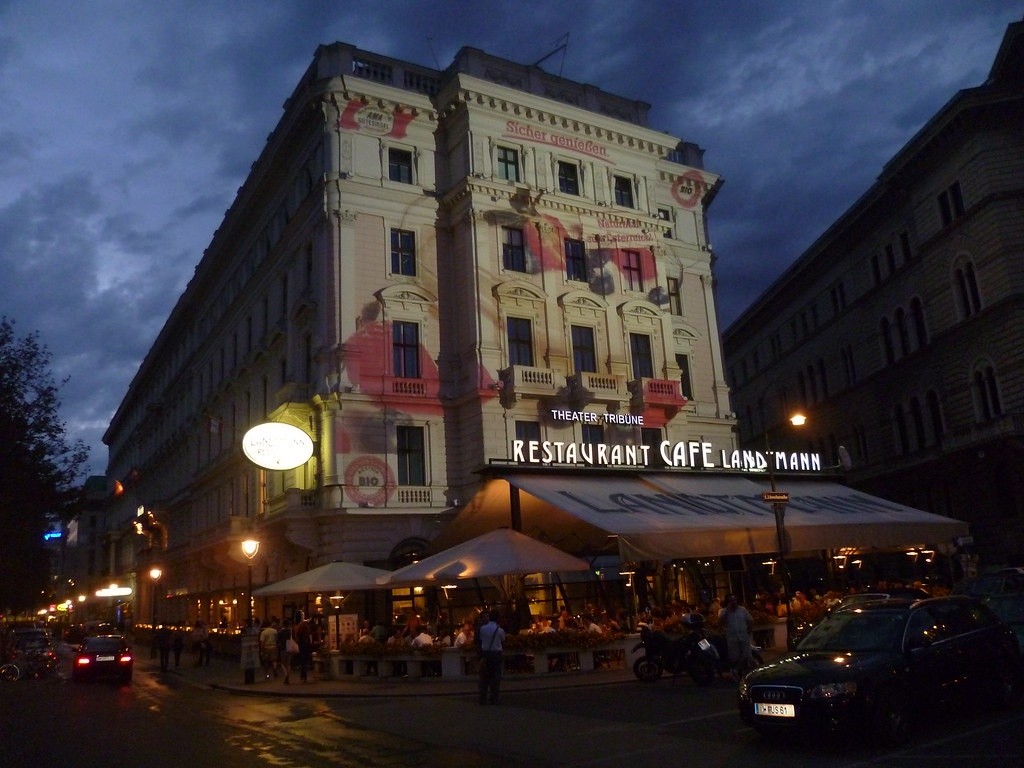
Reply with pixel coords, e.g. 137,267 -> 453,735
286,639 -> 300,654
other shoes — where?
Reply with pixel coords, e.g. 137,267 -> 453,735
479,657 -> 488,668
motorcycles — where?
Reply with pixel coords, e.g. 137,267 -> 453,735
630,612 -> 730,687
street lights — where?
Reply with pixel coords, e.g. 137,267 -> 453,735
240,525 -> 261,685
148,563 -> 163,659
762,412 -> 807,652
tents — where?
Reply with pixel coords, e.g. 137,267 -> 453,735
427,470 -> 967,633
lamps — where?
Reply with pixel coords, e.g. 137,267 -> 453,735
239,527 -> 261,560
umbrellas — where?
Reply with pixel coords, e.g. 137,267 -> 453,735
392,528 -> 591,613
251,560 -> 392,649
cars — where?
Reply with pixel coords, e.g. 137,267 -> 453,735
737,587 -> 1024,749
71,633 -> 134,684
0,620 -> 62,685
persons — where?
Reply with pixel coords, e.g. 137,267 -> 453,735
155,552 -> 965,706
